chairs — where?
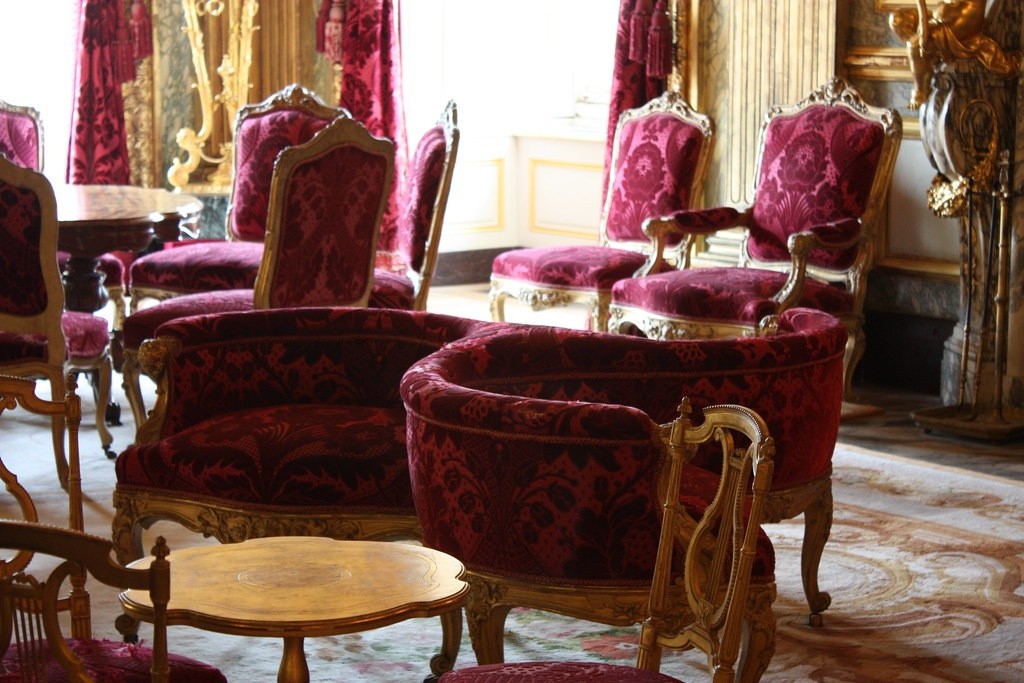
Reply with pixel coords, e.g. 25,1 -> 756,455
0,72 -> 906,683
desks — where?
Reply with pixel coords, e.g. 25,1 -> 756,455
53,184 -> 205,426
114,535 -> 472,683
